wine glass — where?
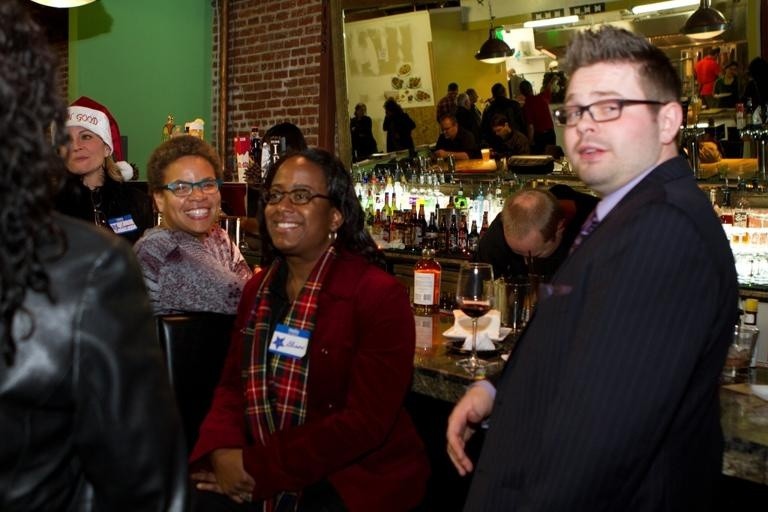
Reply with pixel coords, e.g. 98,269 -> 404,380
455,262 -> 495,366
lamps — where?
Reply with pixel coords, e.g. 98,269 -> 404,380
472,0 -> 514,64
680,0 -> 728,39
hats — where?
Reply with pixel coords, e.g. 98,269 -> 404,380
50,96 -> 133,181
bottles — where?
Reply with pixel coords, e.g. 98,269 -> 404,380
467,220 -> 479,256
736,96 -> 761,192
380,192 -> 391,244
453,191 -> 466,209
681,112 -> 705,180
365,204 -> 374,242
457,215 -> 467,254
447,214 -> 457,253
432,204 -> 439,229
389,193 -> 398,224
353,167 -> 505,204
406,202 -> 417,251
506,181 -> 516,198
762,125 -> 768,188
540,176 -> 551,191
373,210 -> 382,229
414,204 -> 426,250
248,126 -> 262,181
735,298 -> 760,378
425,212 -> 437,252
363,208 -> 368,226
437,215 -> 447,252
478,211 -> 488,246
446,192 -> 455,227
390,210 -> 409,248
531,179 -> 537,191
409,232 -> 441,316
457,201 -> 467,220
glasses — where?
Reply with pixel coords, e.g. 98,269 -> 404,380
554,97 -> 668,127
158,178 -> 223,197
440,127 -> 455,132
262,188 -> 337,205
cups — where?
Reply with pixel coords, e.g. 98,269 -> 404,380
721,325 -> 760,381
480,149 -> 490,162
501,281 -> 530,335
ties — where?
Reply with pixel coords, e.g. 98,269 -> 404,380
564,207 -> 601,253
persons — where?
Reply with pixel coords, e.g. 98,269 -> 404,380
492,114 -> 530,159
51,96 -> 147,246
520,75 -> 561,154
429,114 -> 478,162
712,61 -> 744,107
0,0 -> 185,512
437,83 -> 459,124
479,83 -> 529,138
466,89 -> 481,123
468,185 -> 600,322
694,46 -> 720,108
350,103 -> 378,161
130,136 -> 251,318
251,122 -> 394,277
740,57 -> 768,112
545,144 -> 564,159
383,97 -> 417,164
454,93 -> 478,145
186,150 -> 429,512
446,26 -> 739,512
